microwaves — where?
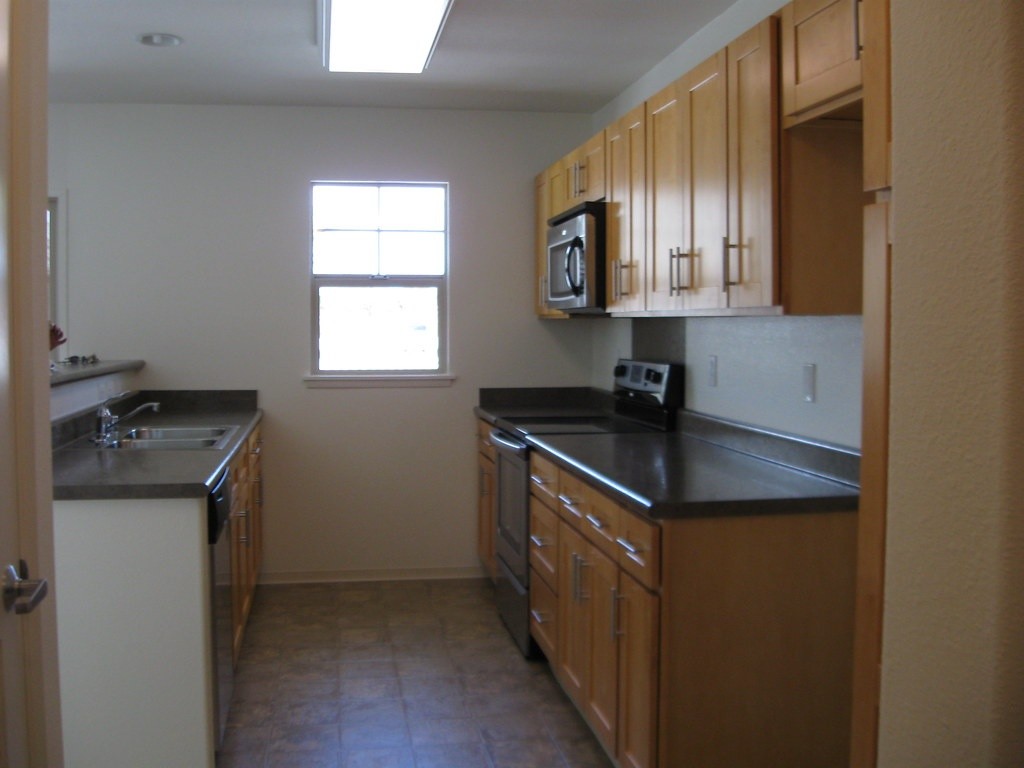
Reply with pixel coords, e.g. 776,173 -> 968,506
543,213 -> 604,309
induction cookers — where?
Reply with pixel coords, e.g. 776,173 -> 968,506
494,359 -> 684,439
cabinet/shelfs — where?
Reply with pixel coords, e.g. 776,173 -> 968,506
534,0 -> 863,319
229,423 -> 263,670
527,450 -> 860,768
475,418 -> 501,586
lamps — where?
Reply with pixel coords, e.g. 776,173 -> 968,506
322,0 -> 455,74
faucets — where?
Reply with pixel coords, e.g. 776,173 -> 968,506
95,390 -> 161,445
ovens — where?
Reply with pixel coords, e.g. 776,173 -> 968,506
489,428 -> 531,659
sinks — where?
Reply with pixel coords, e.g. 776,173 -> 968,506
104,440 -> 220,449
119,427 -> 229,440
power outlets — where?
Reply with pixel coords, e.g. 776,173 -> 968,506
707,355 -> 716,386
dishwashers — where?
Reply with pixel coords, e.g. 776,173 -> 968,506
206,464 -> 235,754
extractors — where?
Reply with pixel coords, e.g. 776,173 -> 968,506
561,308 -> 613,320
